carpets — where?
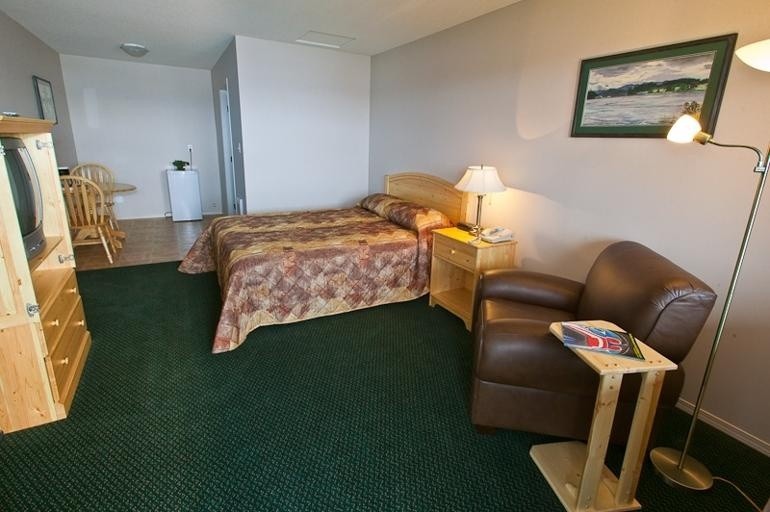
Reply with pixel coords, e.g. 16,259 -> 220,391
0,261 -> 770,511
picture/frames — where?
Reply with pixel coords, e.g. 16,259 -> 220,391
569,33 -> 738,140
32,75 -> 60,126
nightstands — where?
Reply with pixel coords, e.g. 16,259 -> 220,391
427,229 -> 517,336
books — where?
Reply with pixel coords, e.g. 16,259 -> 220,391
561,321 -> 645,362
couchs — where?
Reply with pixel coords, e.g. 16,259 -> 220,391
468,241 -> 717,447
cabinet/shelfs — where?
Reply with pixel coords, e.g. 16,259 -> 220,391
1,115 -> 93,435
167,170 -> 202,222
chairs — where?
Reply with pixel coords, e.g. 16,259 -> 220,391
70,163 -> 120,240
60,174 -> 119,265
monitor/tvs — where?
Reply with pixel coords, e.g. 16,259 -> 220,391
0,137 -> 46,260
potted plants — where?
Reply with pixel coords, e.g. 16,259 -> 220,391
172,160 -> 189,170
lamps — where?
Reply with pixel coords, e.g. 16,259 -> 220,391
454,163 -> 507,236
651,38 -> 770,491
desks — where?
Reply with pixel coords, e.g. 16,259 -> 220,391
528,320 -> 676,512
62,183 -> 138,239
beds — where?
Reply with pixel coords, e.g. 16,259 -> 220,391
176,174 -> 468,355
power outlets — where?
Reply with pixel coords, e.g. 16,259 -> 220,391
187,145 -> 193,153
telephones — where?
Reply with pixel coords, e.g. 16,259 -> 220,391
468,227 -> 513,245
